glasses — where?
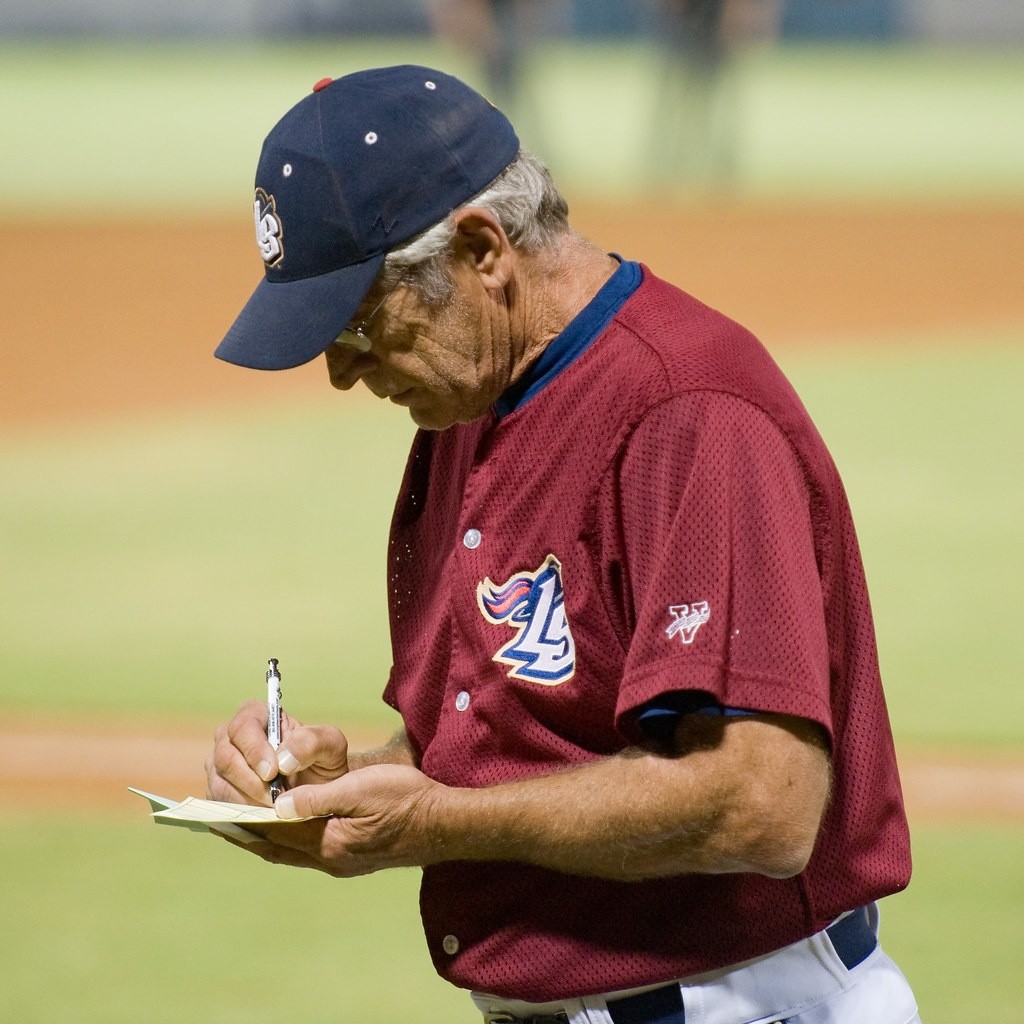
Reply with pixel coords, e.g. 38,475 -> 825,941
322,264 -> 419,356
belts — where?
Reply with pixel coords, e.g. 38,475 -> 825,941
483,907 -> 879,1023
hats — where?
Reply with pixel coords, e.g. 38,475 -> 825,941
205,62 -> 519,374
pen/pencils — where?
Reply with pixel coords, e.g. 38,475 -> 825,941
261,655 -> 285,809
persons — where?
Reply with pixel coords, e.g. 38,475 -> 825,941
200,68 -> 929,1024
